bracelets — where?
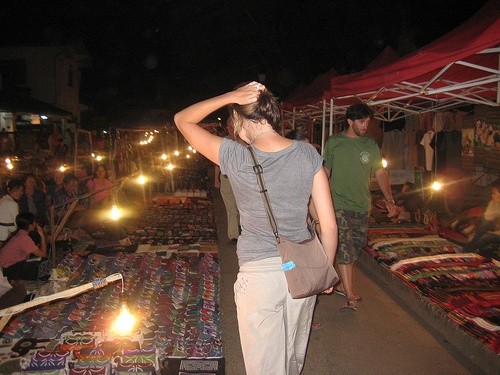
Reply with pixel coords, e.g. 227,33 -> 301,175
386,199 -> 395,205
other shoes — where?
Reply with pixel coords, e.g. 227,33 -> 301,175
231,238 -> 237,244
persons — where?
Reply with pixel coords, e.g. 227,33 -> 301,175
376,181 -> 424,223
0,212 -> 54,281
320,101 -> 400,311
1,126 -> 113,260
173,78 -> 340,374
462,177 -> 500,255
214,115 -> 252,244
1,286 -> 36,308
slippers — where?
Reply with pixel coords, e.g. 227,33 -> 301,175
342,302 -> 357,311
335,289 -> 360,300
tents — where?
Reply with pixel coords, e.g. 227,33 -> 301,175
1,96 -> 74,134
279,0 -> 500,168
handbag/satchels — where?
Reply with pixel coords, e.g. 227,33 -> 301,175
277,224 -> 339,299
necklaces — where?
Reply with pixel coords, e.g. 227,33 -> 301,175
250,129 -> 277,143
